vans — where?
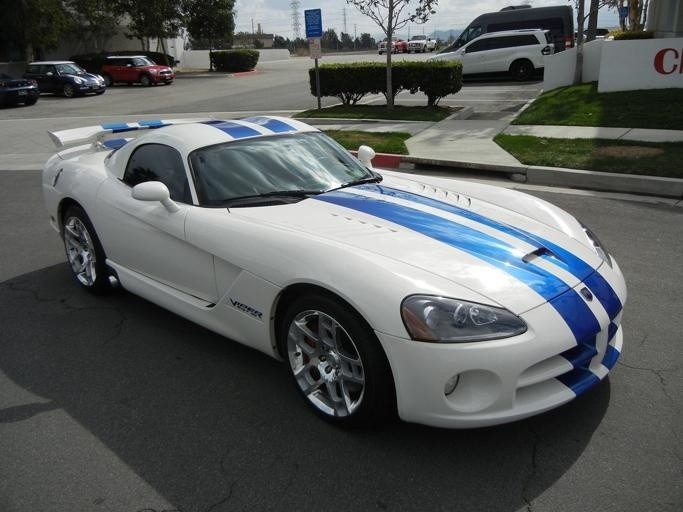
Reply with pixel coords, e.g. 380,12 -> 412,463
439,5 -> 573,56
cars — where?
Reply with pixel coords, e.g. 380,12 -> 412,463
0,72 -> 40,106
23,60 -> 106,97
100,55 -> 176,86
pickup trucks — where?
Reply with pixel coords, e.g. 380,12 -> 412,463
378,35 -> 408,55
406,34 -> 436,53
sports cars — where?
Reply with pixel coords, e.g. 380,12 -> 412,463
40,116 -> 628,428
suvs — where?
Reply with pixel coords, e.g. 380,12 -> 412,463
425,28 -> 556,81
574,28 -> 609,41
